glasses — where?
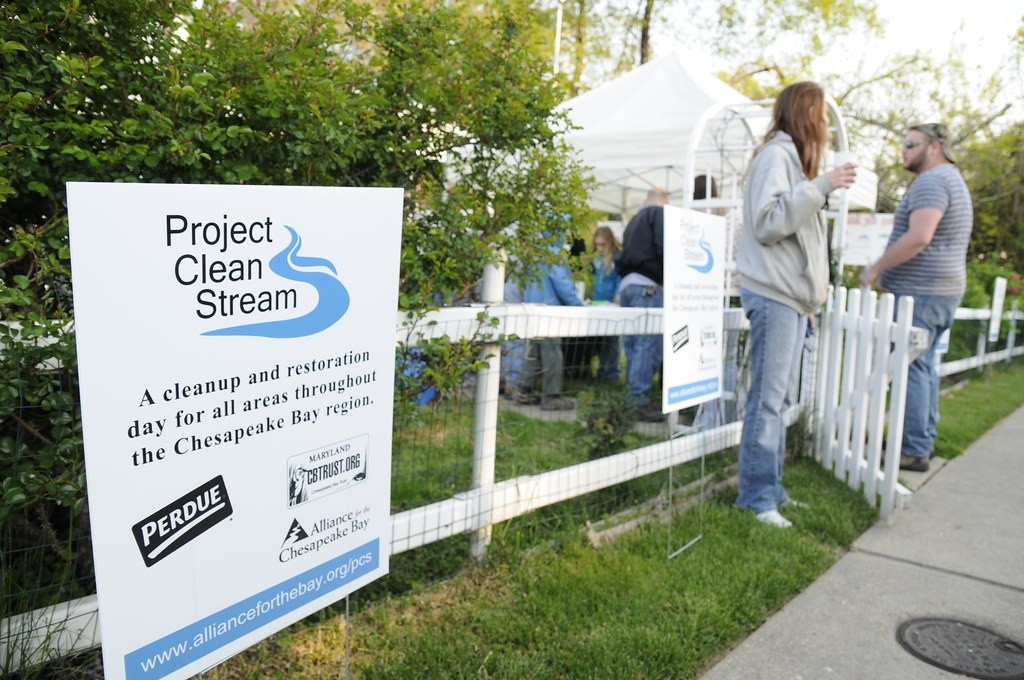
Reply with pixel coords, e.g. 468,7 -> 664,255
595,241 -> 607,247
902,140 -> 930,148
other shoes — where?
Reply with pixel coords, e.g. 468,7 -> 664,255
883,434 -> 937,471
516,386 -> 574,412
628,395 -> 665,422
751,485 -> 811,528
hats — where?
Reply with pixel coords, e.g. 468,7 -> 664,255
917,123 -> 955,164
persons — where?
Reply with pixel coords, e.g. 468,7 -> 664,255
614,186 -> 669,422
737,81 -> 858,529
500,200 -> 624,411
860,123 -> 973,472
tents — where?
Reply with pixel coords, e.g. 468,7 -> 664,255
442,48 -> 876,213
